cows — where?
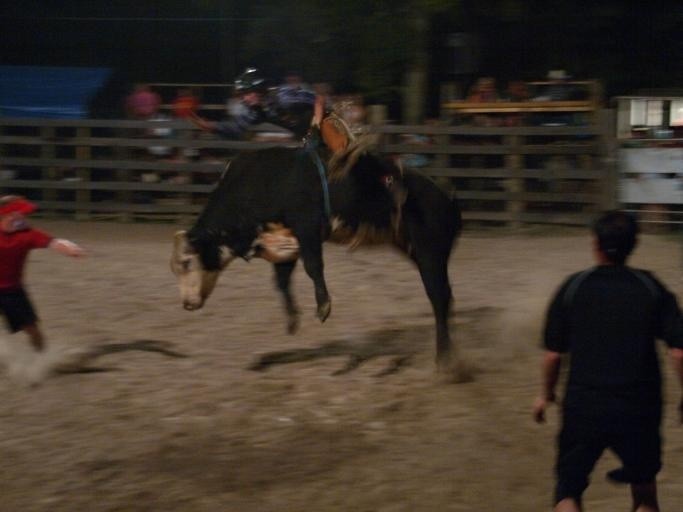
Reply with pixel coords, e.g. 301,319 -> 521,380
167,145 -> 464,373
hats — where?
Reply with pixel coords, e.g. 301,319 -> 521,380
0,195 -> 37,215
471,77 -> 497,92
544,70 -> 572,79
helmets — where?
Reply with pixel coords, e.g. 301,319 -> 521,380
230,66 -> 269,96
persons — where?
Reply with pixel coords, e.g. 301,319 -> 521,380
0,194 -> 87,358
187,66 -> 375,250
529,207 -> 681,511
45,64 -> 683,232
